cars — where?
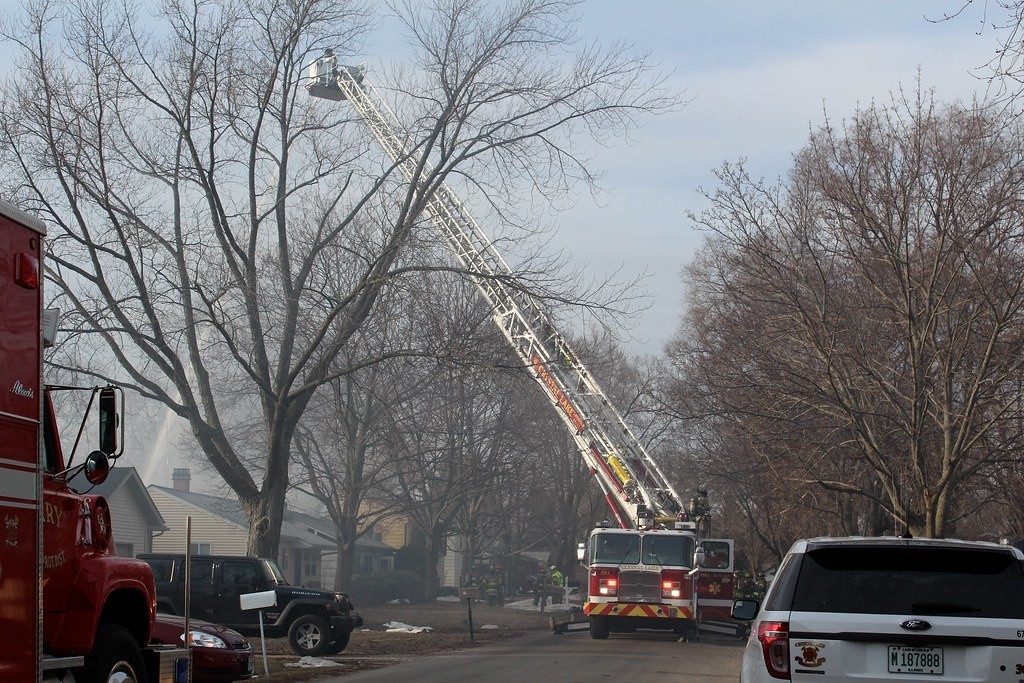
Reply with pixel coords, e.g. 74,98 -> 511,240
149,613 -> 255,683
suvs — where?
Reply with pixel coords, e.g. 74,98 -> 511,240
728,531 -> 1024,683
132,552 -> 364,659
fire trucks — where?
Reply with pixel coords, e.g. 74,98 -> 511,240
304,54 -> 754,642
1,195 -> 157,683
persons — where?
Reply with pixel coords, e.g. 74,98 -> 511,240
465,564 -> 506,607
733,570 -> 767,604
687,486 -> 710,538
530,564 -> 564,607
324,49 -> 339,77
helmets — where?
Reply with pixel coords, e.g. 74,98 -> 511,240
697,484 -> 708,496
550,565 -> 556,570
758,573 -> 765,577
